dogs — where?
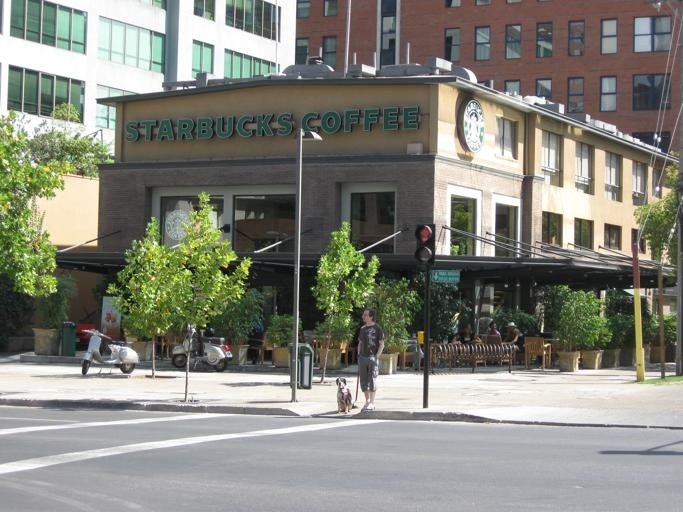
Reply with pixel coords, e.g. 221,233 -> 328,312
336,377 -> 358,415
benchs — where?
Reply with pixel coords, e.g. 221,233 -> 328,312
430,343 -> 517,368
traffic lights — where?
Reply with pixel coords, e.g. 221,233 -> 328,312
414,223 -> 435,266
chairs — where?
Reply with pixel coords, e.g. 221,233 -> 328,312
525,336 -> 553,368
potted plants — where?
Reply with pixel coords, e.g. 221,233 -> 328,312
118,277 -> 422,374
542,282 -> 677,371
34,278 -> 75,356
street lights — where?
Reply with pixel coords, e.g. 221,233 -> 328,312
288,126 -> 324,401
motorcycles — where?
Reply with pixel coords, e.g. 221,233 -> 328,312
80,328 -> 139,377
169,323 -> 231,372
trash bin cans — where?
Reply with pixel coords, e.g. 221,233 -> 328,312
288,342 -> 314,389
62,321 -> 76,356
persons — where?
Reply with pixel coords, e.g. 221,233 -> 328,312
355,308 -> 385,412
451,321 -> 483,345
500,322 -> 526,365
483,320 -> 502,343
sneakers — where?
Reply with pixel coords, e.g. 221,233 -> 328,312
366,403 -> 375,411
361,402 -> 369,413
